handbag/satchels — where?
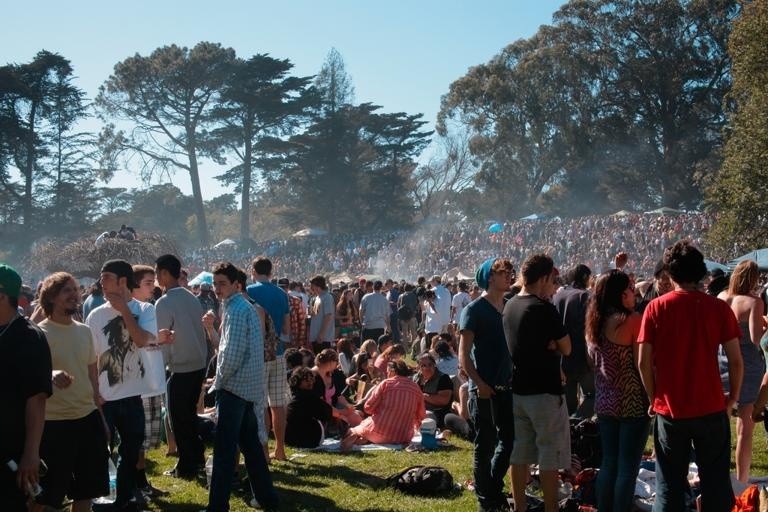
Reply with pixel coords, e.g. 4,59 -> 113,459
398,304 -> 411,320
357,379 -> 373,398
395,465 -> 457,497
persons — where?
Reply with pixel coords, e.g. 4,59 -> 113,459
0,212 -> 768,512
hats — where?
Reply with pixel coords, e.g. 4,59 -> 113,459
475,258 -> 496,289
374,281 -> 382,288
187,270 -> 214,286
427,275 -> 441,282
0,265 -> 22,298
101,260 -> 141,289
277,278 -> 289,287
654,260 -> 664,277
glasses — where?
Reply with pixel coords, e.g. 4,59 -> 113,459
491,270 -> 515,279
304,378 -> 312,381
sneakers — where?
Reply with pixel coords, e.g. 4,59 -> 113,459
130,490 -> 153,505
137,485 -> 169,497
